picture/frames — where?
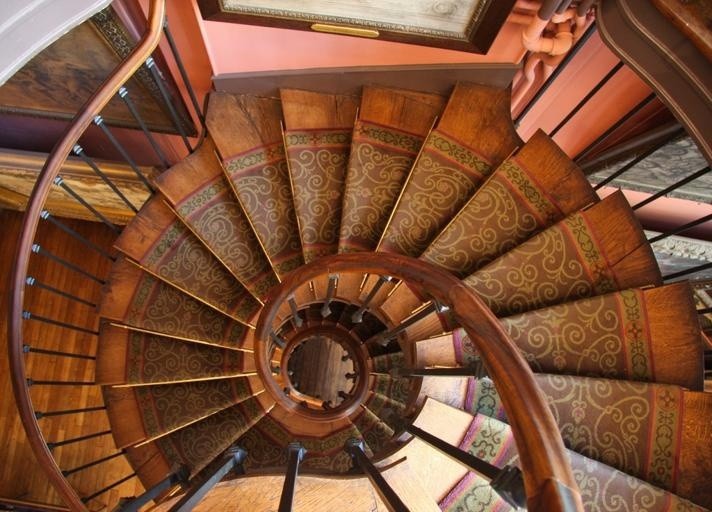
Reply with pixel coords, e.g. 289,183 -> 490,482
196,0 -> 518,55
577,121 -> 711,290
